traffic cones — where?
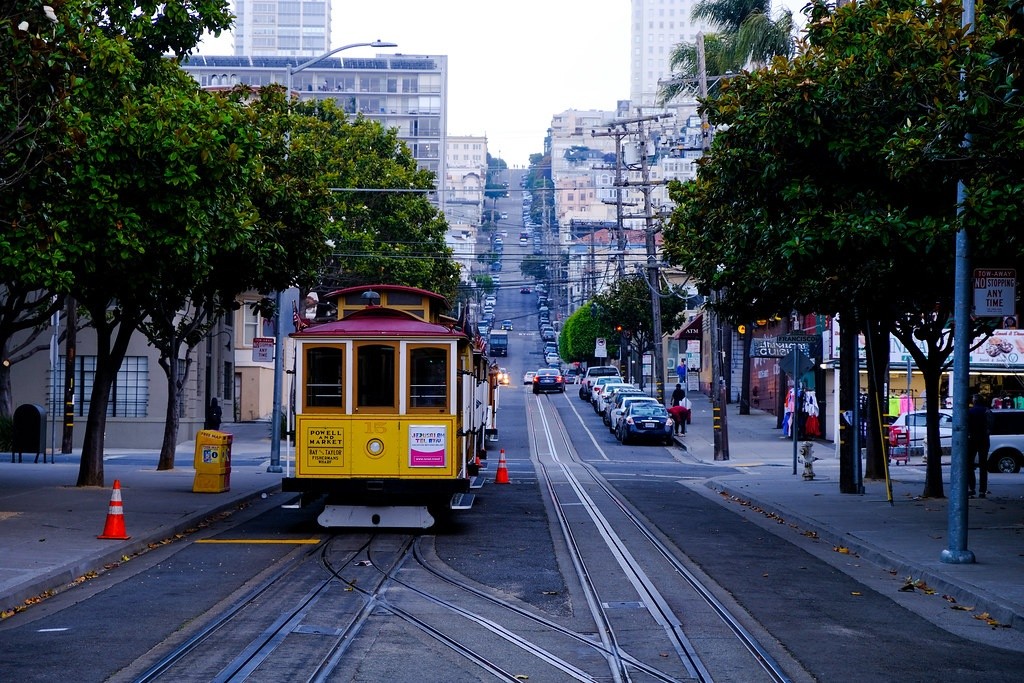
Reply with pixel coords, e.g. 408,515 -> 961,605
493,449 -> 512,485
97,479 -> 132,540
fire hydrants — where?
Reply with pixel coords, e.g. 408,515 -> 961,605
797,441 -> 819,481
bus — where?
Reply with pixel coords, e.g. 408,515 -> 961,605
281,282 -> 500,513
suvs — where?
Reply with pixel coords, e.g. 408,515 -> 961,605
609,396 -> 660,433
890,409 -> 953,454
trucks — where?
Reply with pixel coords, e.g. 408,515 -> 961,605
486,329 -> 508,357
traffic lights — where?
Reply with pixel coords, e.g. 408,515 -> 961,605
614,323 -> 627,332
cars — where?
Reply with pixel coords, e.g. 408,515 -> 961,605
590,376 -> 625,408
564,369 -> 576,383
523,372 -> 536,385
519,176 -> 544,255
498,368 -> 511,385
614,403 -> 675,447
594,382 -> 636,416
535,284 -> 561,373
492,181 -> 510,252
520,284 -> 531,294
603,389 -> 649,426
476,262 -> 503,344
974,409 -> 1024,473
579,366 -> 622,403
500,319 -> 514,331
532,369 -> 566,394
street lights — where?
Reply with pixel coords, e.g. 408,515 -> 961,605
266,38 -> 398,474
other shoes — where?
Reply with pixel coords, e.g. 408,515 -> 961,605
679,434 -> 686,437
674,433 -> 679,436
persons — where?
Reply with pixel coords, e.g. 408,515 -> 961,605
968,395 -> 992,499
667,406 -> 688,436
676,361 -> 688,383
669,384 -> 685,406
574,366 -> 587,385
206,397 -> 222,431
